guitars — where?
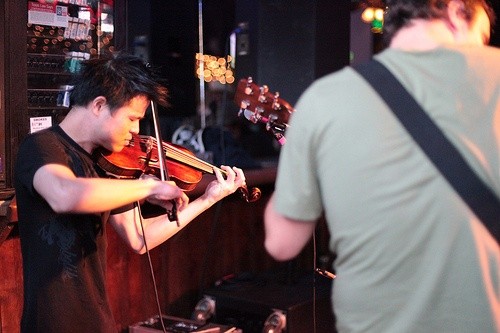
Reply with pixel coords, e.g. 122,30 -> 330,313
235,77 -> 298,133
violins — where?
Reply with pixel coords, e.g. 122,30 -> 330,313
95,131 -> 263,203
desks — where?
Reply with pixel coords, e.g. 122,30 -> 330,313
207,273 -> 338,333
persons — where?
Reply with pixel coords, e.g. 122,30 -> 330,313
264,0 -> 500,333
14,51 -> 246,333
